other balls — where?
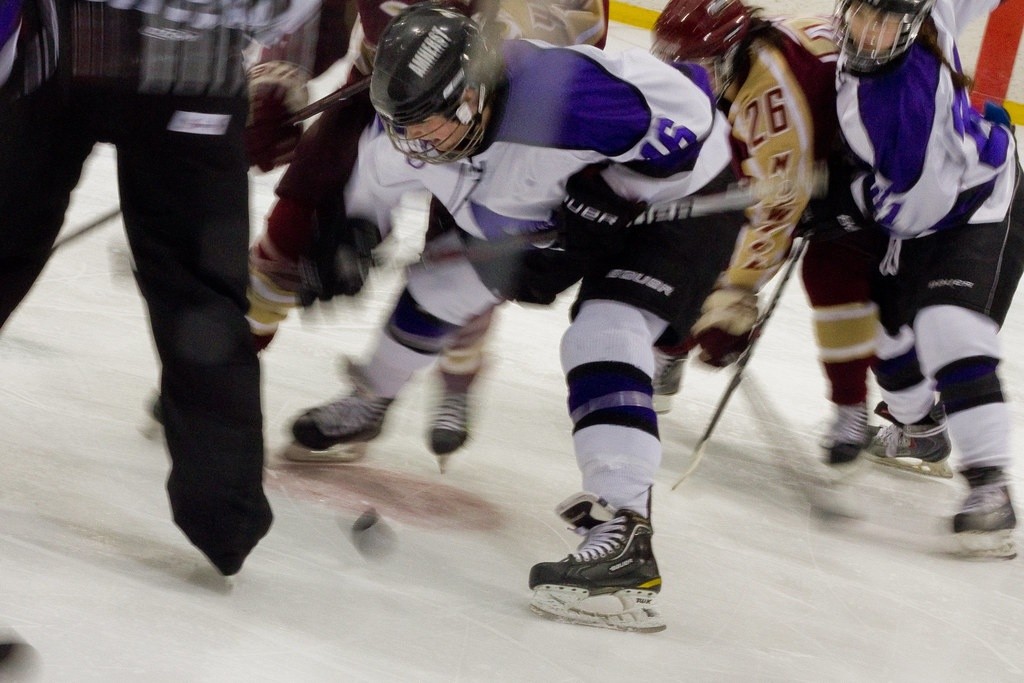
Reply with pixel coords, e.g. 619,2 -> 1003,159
343,504 -> 396,556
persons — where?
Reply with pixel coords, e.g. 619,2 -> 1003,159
830,0 -> 1023,543
0,0 -> 322,589
648,0 -> 888,474
142,0 -> 610,479
275,4 -> 749,600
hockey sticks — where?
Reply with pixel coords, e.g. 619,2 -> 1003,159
667,190 -> 821,489
278,164 -> 833,305
43,68 -> 375,253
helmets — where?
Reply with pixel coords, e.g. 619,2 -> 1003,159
651,0 -> 748,106
832,0 -> 933,74
369,4 -> 496,126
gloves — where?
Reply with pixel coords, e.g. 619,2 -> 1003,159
693,283 -> 761,369
551,161 -> 644,262
295,216 -> 381,307
803,173 -> 877,240
243,60 -> 310,170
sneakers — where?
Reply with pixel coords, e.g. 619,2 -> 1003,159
651,346 -> 689,415
866,401 -> 955,480
945,464 -> 1017,562
697,348 -> 740,375
430,362 -> 481,474
820,395 -> 870,480
526,491 -> 670,631
287,370 -> 395,461
